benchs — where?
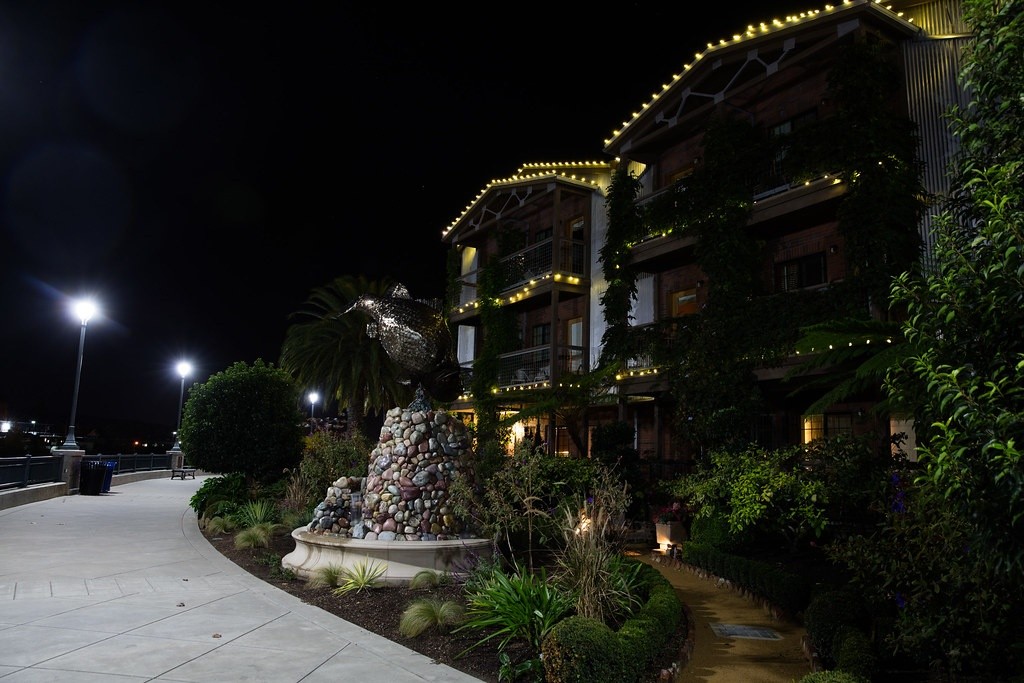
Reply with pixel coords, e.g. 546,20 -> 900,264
171,469 -> 196,480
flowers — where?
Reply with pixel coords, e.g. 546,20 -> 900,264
653,502 -> 688,525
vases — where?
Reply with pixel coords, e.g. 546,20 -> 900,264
652,521 -> 687,554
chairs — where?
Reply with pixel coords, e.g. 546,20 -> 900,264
511,368 -> 549,384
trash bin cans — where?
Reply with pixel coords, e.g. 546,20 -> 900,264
79,460 -> 117,495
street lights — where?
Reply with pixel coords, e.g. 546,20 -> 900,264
166,359 -> 193,470
308,391 -> 319,435
50,292 -> 96,493
